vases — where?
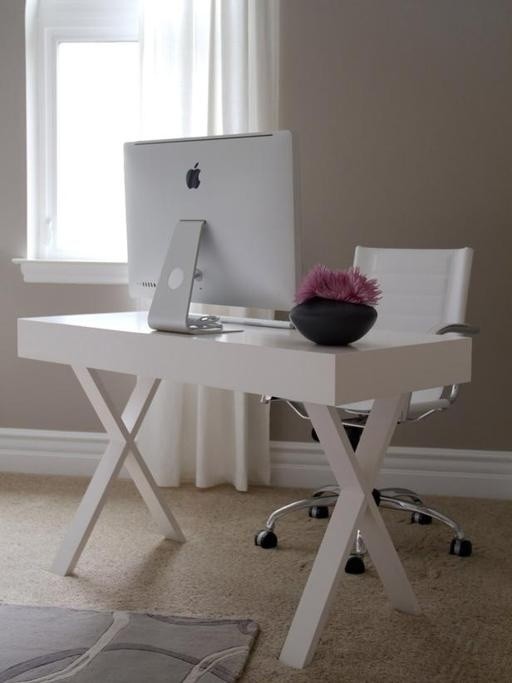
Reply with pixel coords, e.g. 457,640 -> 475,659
292,298 -> 377,347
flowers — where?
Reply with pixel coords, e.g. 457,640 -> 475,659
292,265 -> 382,305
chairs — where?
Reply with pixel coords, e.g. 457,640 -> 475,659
255,247 -> 474,574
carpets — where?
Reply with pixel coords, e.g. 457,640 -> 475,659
0,607 -> 260,683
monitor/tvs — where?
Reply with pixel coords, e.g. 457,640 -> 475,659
122,127 -> 307,334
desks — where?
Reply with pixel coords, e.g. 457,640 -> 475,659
16,311 -> 472,669
